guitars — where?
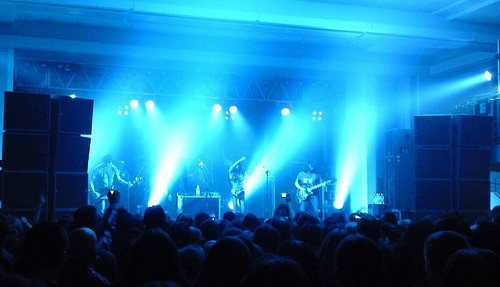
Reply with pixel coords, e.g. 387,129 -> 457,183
297,178 -> 337,201
88,177 -> 144,206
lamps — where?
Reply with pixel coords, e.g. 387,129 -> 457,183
280,102 -> 294,115
130,95 -> 157,109
146,70 -> 211,81
37,61 -> 78,72
285,79 -> 332,88
211,99 -> 237,113
485,66 -> 498,81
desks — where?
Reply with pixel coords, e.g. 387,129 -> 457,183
178,195 -> 219,219
368,204 -> 392,220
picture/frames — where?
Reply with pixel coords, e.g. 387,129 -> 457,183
473,92 -> 494,115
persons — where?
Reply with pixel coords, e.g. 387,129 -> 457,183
295,159 -> 328,218
89,154 -> 132,214
229,156 -> 249,213
308,206 -> 500,287
0,190 -> 312,287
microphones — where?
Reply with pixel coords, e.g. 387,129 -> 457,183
266,170 -> 268,173
120,161 -> 127,167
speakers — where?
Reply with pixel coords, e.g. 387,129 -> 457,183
369,114 -> 496,221
0,91 -> 95,225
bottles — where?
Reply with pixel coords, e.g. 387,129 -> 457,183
374,193 -> 385,204
196,185 -> 200,196
